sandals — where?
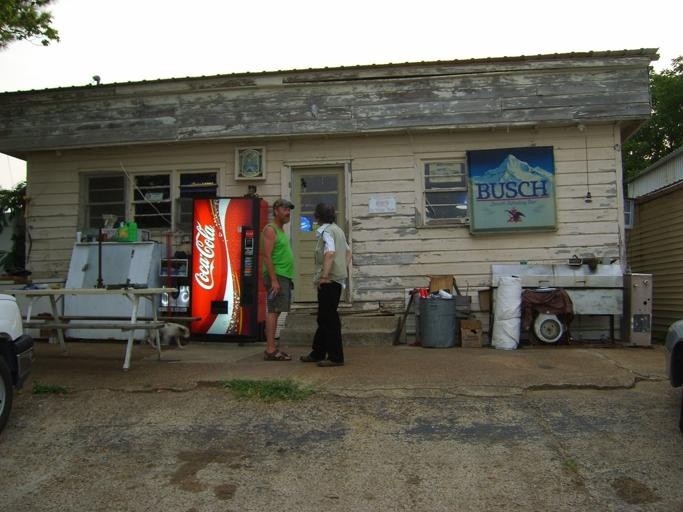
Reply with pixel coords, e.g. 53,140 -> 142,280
264,350 -> 291,360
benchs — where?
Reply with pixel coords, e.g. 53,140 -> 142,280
5,287 -> 201,372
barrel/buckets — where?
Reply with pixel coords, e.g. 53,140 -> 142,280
419,298 -> 459,347
118,221 -> 137,242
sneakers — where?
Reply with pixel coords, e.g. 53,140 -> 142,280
301,355 -> 344,367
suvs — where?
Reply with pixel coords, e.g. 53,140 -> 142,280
0,292 -> 37,434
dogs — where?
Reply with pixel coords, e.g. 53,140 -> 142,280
145,320 -> 191,350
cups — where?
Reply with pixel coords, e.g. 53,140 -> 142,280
76,231 -> 82,243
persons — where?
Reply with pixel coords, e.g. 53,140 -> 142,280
259,198 -> 294,362
297,201 -> 353,368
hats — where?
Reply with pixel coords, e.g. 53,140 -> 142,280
274,198 -> 295,209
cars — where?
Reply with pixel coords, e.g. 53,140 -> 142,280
664,317 -> 683,431
